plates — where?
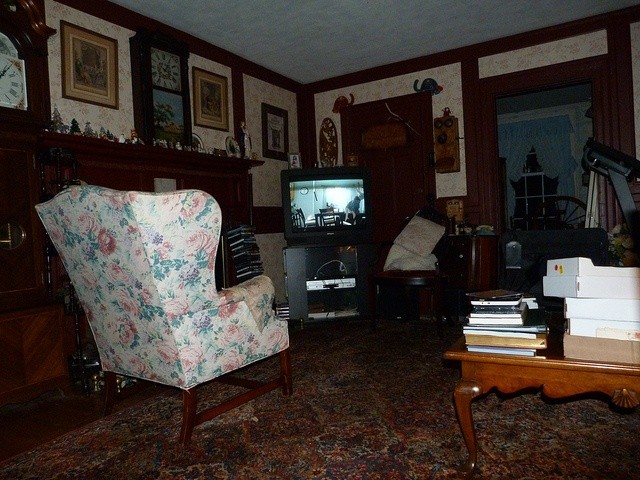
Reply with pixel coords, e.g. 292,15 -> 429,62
225,136 -> 242,159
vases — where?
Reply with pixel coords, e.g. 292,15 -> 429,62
615,244 -> 625,266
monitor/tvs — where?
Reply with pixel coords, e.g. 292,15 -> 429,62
279,167 -> 379,248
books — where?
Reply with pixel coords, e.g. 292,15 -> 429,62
463,289 -> 553,362
229,223 -> 291,321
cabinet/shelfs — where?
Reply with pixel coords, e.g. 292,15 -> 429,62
0,126 -> 73,405
40,131 -> 265,386
431,234 -> 498,319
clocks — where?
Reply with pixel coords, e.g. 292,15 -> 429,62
0,54 -> 26,110
151,47 -> 182,91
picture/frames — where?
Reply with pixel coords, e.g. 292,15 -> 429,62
60,20 -> 119,110
261,102 -> 289,161
192,65 -> 229,132
288,153 -> 303,170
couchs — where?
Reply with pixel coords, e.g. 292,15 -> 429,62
498,226 -> 613,292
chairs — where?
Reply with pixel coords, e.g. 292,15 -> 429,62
368,207 -> 450,335
35,185 -> 293,445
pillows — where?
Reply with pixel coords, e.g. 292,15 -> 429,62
393,216 -> 446,257
384,244 -> 438,271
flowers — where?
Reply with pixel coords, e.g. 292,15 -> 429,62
608,223 -> 633,258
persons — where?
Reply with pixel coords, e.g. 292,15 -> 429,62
345,193 -> 364,225
239,120 -> 246,159
243,124 -> 251,158
291,156 -> 298,167
